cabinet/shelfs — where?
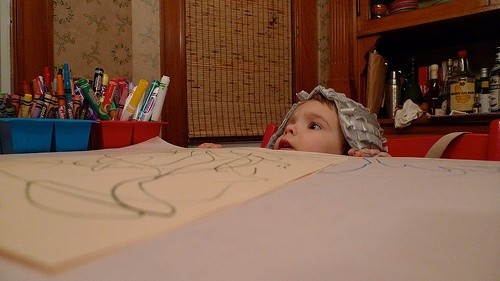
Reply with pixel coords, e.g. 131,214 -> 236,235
329,0 -> 500,135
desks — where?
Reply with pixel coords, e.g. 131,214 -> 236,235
0,136 -> 500,281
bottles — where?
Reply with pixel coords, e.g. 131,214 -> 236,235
388,46 -> 500,117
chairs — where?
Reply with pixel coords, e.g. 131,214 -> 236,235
260,119 -> 500,162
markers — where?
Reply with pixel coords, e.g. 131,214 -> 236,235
0,62 -> 169,121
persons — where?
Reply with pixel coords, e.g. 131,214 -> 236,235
198,83 -> 395,159
